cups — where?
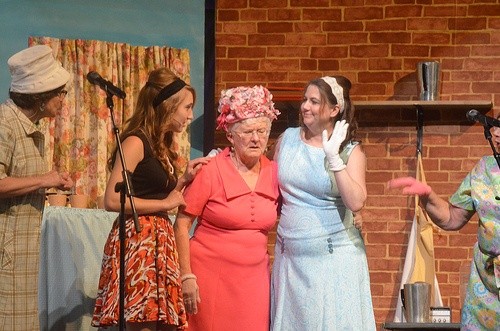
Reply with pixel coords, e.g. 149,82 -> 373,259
70,195 -> 89,208
47,195 -> 70,207
97,196 -> 105,209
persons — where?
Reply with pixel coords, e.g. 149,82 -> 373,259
172,84 -> 361,331
0,45 -> 75,331
388,109 -> 500,331
91,68 -> 212,331
217,74 -> 376,331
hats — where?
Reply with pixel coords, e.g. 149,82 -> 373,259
8,45 -> 70,93
217,85 -> 282,130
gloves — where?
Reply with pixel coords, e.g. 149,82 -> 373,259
322,119 -> 349,171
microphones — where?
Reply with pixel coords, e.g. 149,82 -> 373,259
86,72 -> 127,99
467,110 -> 500,127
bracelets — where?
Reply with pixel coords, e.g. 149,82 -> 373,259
181,273 -> 197,283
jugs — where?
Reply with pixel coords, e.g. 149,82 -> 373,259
400,281 -> 433,323
415,61 -> 441,101
